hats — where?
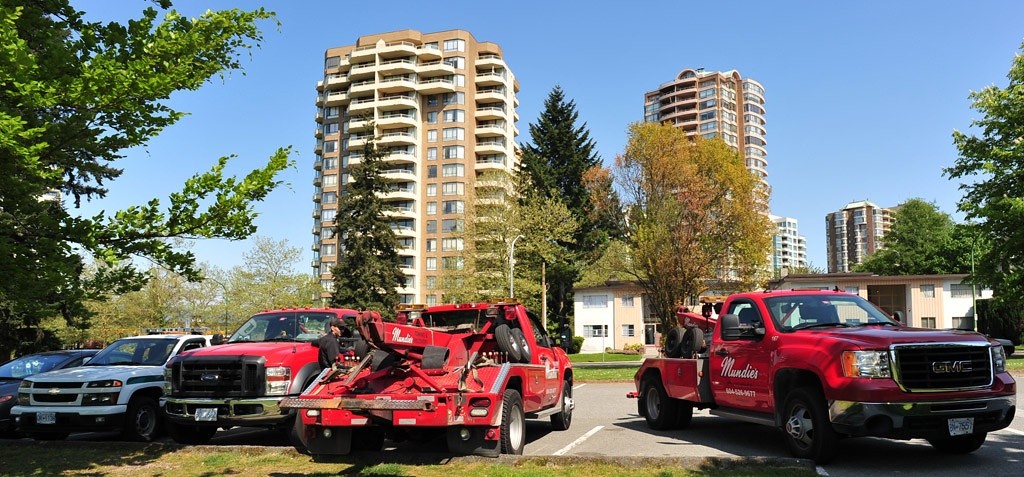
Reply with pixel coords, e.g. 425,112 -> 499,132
330,318 -> 349,327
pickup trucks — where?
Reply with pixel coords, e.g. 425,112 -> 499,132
290,302 -> 579,455
630,294 -> 1015,459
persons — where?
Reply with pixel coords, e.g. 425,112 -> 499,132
318,318 -> 349,374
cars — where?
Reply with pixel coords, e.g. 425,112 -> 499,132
11,335 -> 234,444
0,351 -> 142,429
164,306 -> 387,438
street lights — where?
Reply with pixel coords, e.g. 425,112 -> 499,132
509,234 -> 525,298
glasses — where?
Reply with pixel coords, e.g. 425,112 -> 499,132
337,325 -> 345,330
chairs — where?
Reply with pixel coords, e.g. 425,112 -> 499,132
799,304 -> 839,325
145,345 -> 165,364
738,307 -> 759,324
